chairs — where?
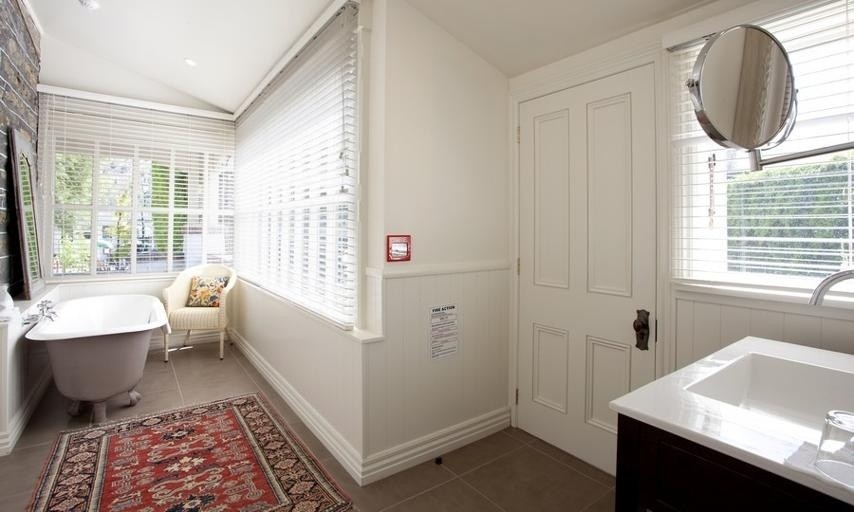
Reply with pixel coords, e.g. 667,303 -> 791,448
161,263 -> 237,362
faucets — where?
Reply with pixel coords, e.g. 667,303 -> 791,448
808,268 -> 854,307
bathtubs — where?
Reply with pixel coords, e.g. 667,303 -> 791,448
23,292 -> 168,405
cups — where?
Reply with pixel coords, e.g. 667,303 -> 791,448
814,410 -> 854,489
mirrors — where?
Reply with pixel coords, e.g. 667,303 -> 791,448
685,23 -> 798,151
8,127 -> 46,300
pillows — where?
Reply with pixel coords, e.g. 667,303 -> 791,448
186,275 -> 230,307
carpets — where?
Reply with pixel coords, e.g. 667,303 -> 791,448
24,391 -> 360,512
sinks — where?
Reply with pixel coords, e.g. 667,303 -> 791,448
683,352 -> 854,434
